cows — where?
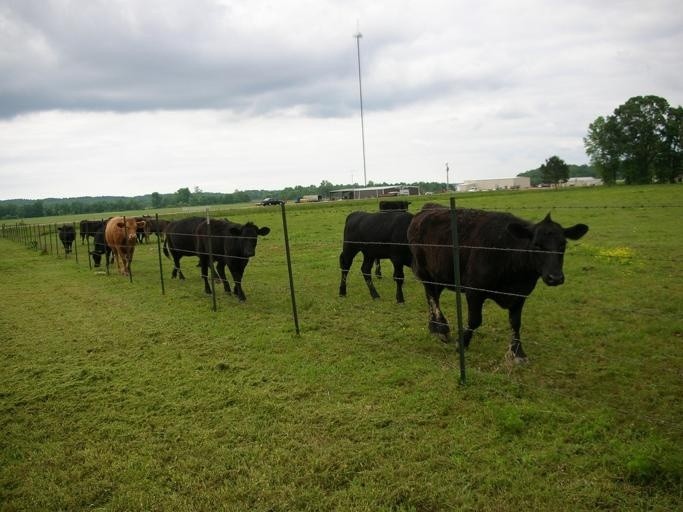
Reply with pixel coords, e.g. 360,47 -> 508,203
378,199 -> 412,212
79,214 -> 169,277
56,224 -> 77,254
162,216 -> 222,285
338,209 -> 418,305
406,203 -> 589,371
194,218 -> 271,303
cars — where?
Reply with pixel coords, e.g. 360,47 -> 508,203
261,198 -> 285,206
